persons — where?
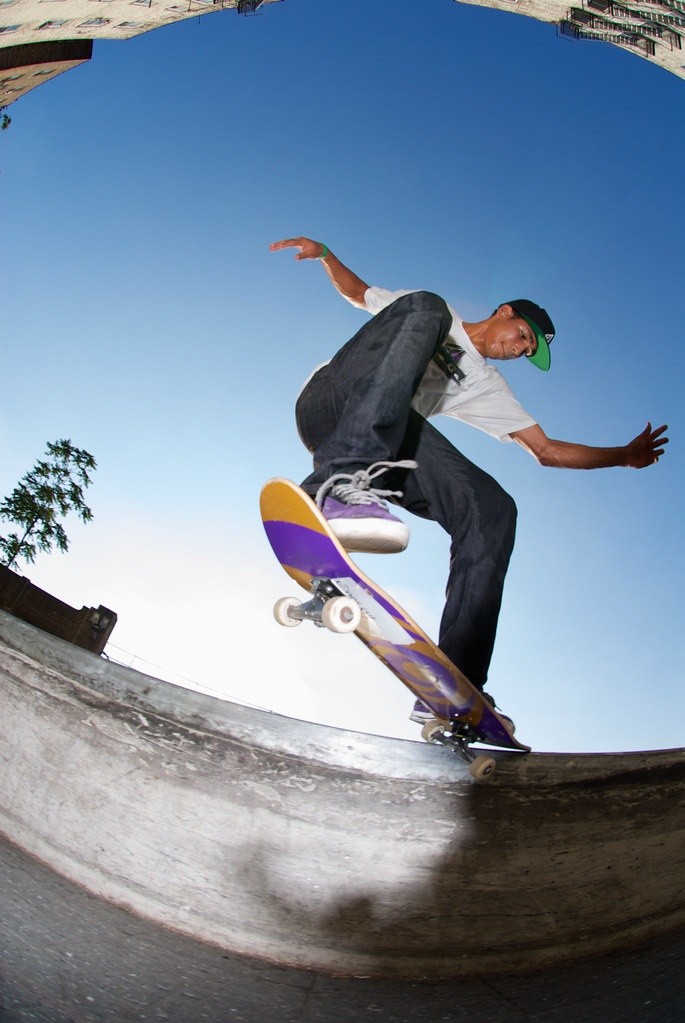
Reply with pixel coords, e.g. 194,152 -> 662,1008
269,238 -> 669,737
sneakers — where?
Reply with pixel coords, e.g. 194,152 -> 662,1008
410,698 -> 515,735
314,460 -> 418,553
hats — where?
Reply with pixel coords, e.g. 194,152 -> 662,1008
493,299 -> 557,371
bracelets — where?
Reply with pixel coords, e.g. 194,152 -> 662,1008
319,242 -> 328,259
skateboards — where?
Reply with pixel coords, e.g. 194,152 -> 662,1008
258,476 -> 534,782
